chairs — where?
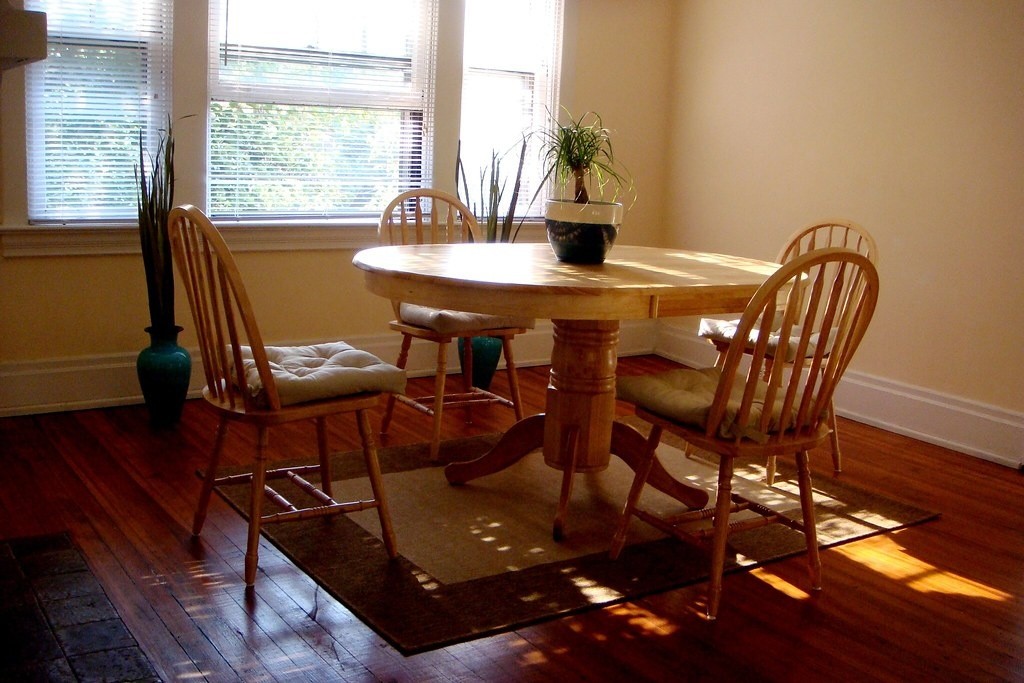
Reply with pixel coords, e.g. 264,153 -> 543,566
376,188 -> 526,461
165,204 -> 411,587
682,218 -> 880,485
610,244 -> 881,620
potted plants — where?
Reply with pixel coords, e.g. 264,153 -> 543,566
454,133 -> 556,390
532,102 -> 636,266
130,110 -> 201,432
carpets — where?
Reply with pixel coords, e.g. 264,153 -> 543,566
195,415 -> 943,658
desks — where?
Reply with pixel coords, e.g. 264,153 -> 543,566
352,240 -> 812,541
